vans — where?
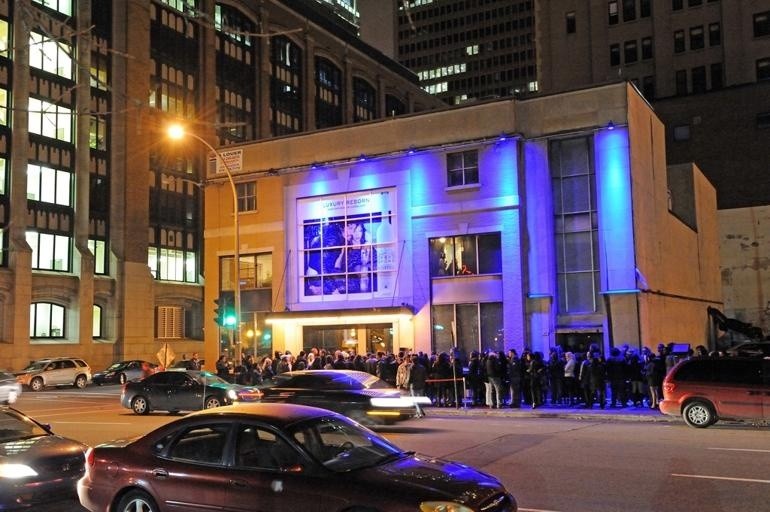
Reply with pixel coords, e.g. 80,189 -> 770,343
655,358 -> 770,426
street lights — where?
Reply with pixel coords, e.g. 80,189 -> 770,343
165,125 -> 244,375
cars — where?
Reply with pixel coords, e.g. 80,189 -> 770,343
120,369 -> 263,415
230,369 -> 421,428
1,370 -> 22,408
75,403 -> 517,512
14,354 -> 206,390
0,404 -> 95,511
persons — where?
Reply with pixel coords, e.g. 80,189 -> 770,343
141,360 -> 153,378
257,444 -> 300,473
303,426 -> 344,479
305,218 -> 378,294
438,252 -> 474,275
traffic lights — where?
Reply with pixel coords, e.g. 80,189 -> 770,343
212,298 -> 220,324
226,301 -> 235,327
